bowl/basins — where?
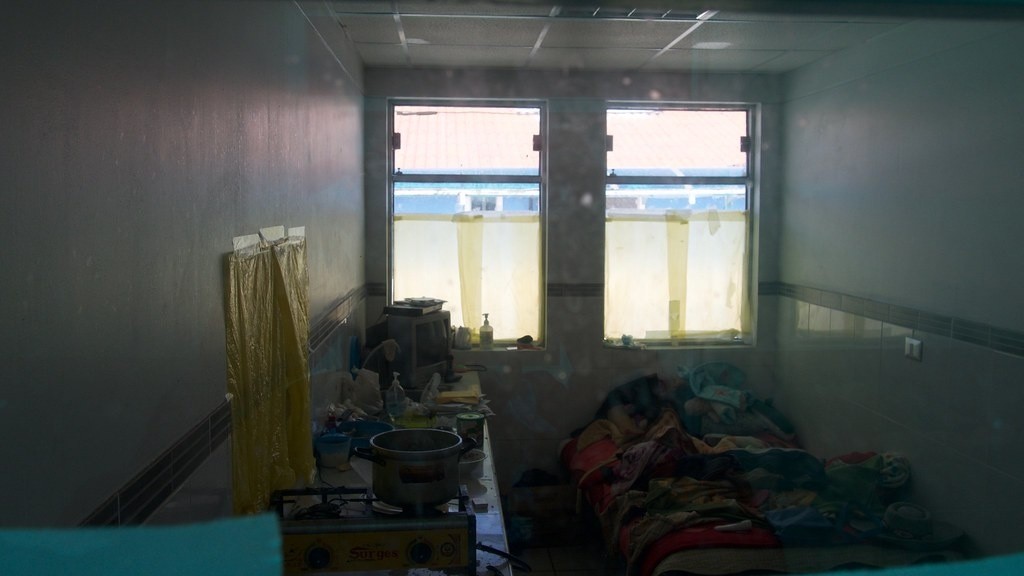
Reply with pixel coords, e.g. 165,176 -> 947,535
459,447 -> 488,479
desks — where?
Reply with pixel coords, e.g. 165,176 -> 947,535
275,369 -> 513,576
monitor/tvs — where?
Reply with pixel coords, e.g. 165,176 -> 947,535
365,310 -> 452,388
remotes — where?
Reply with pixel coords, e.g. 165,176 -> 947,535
444,375 -> 464,384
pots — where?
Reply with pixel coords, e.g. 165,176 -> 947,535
354,427 -> 477,512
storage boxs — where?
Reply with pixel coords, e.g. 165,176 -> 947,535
500,480 -> 579,547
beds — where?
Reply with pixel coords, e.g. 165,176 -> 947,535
558,424 -> 981,576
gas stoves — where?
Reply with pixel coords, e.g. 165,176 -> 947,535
267,486 -> 478,576
